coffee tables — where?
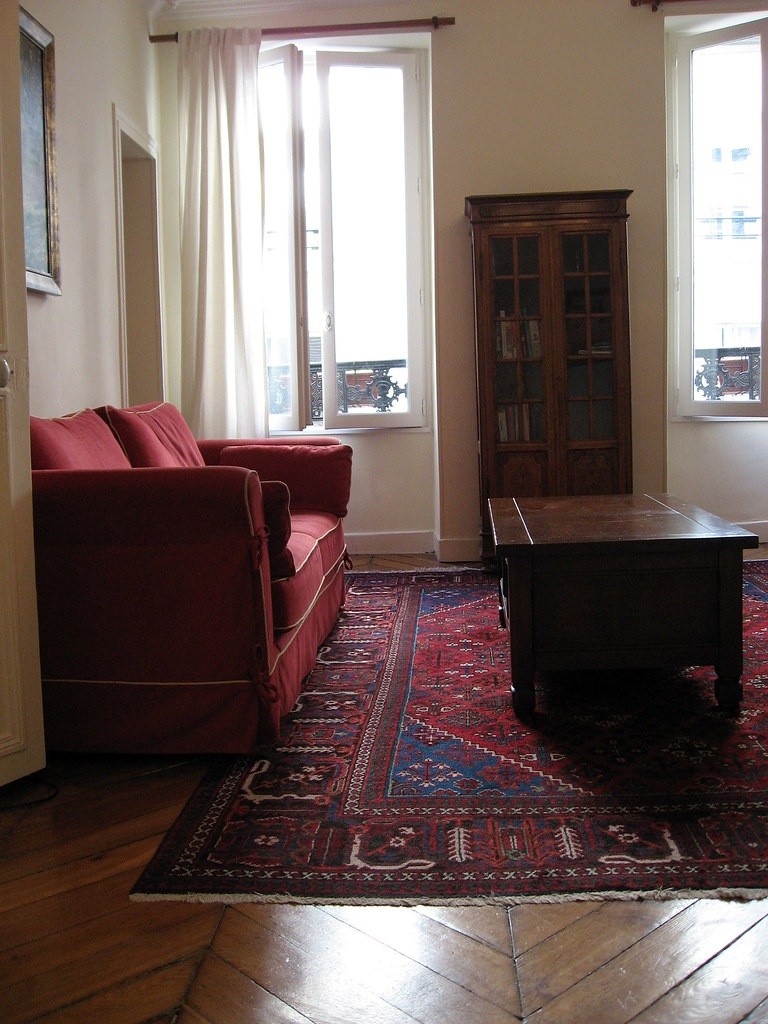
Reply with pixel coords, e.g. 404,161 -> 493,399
487,493 -> 759,720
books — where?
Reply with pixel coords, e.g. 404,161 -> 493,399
494,308 -> 541,359
577,346 -> 612,356
497,402 -> 530,443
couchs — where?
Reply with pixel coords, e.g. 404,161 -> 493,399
29,400 -> 355,753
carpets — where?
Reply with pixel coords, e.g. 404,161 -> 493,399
128,560 -> 768,902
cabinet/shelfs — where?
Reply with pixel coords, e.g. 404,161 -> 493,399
463,189 -> 635,574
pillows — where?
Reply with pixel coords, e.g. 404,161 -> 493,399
261,480 -> 296,578
220,444 -> 353,518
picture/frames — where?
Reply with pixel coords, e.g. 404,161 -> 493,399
17,4 -> 63,297
